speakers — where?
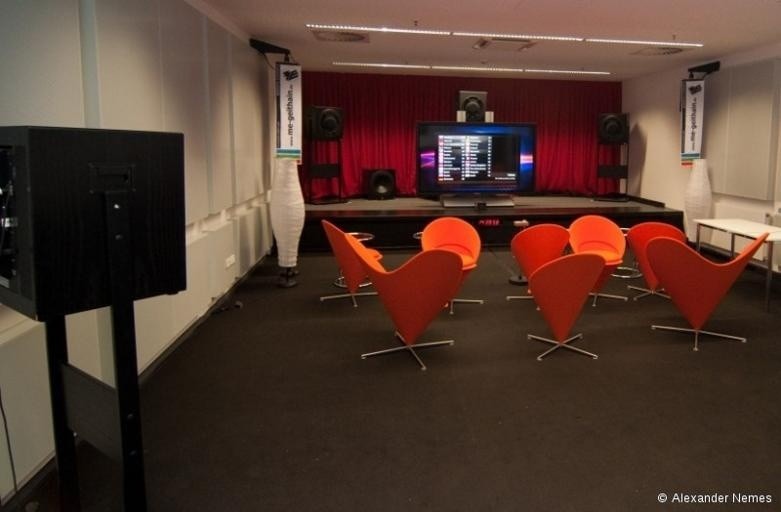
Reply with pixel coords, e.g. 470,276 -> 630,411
367,169 -> 395,200
598,113 -> 630,144
459,90 -> 488,122
0,126 -> 186,321
305,105 -> 344,141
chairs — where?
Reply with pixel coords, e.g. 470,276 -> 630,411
421,217 -> 485,315
628,221 -> 687,300
527,251 -> 607,361
344,233 -> 464,371
320,218 -> 379,307
650,232 -> 772,351
506,223 -> 571,311
569,215 -> 630,307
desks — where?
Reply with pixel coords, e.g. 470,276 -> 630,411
336,232 -> 375,288
696,218 -> 781,312
611,227 -> 644,279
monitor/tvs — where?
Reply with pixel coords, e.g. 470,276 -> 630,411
417,122 -> 538,207
